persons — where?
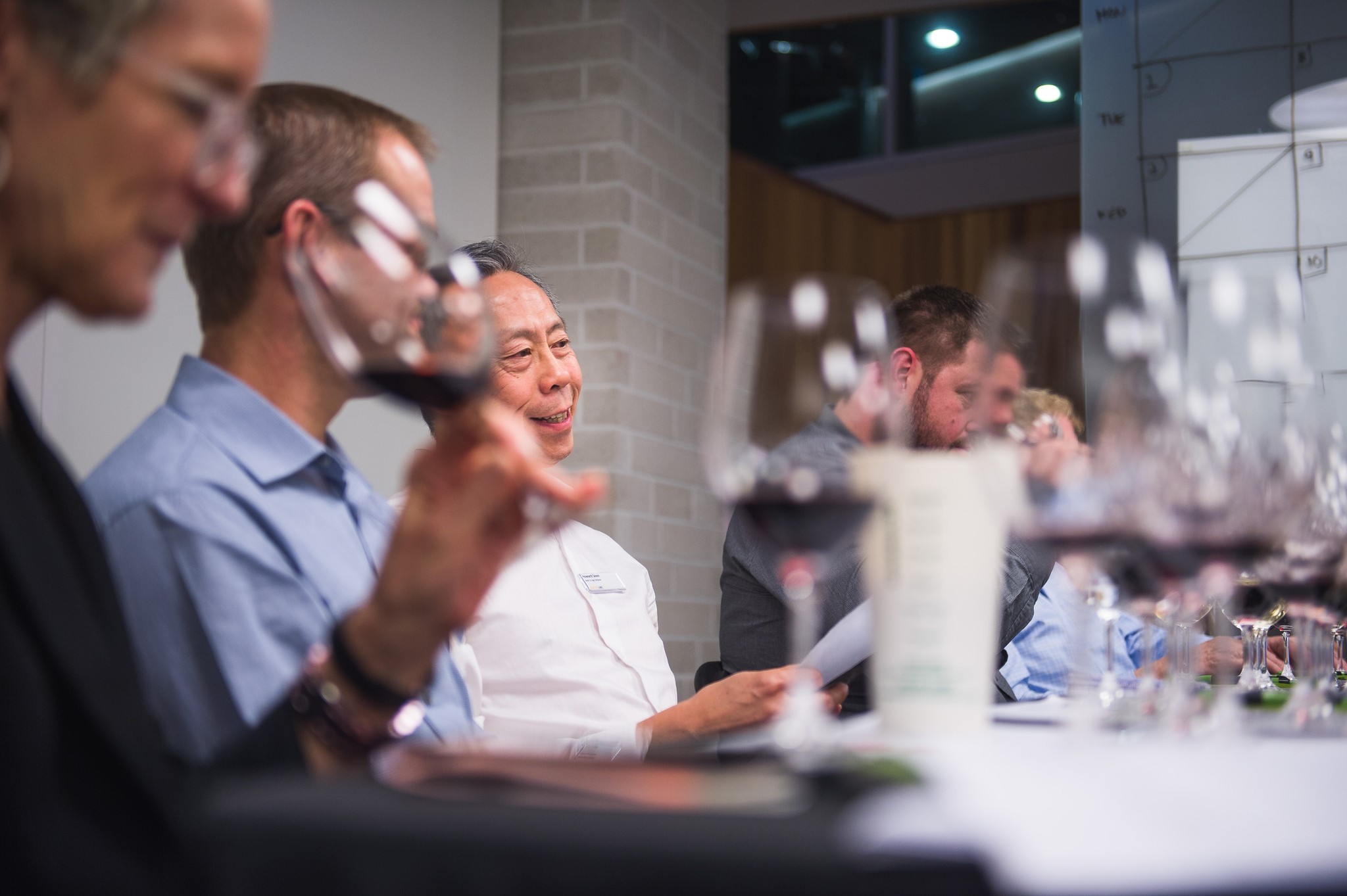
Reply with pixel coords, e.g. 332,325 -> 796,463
0,0 -> 275,896
717,284 -> 1347,723
386,239 -> 847,769
86,80 -> 492,756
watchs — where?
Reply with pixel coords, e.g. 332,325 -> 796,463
283,667 -> 374,769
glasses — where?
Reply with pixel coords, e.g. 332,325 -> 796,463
57,28 -> 264,187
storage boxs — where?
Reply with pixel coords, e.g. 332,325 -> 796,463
1175,130 -> 1347,439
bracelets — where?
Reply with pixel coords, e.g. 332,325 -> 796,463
323,615 -> 418,717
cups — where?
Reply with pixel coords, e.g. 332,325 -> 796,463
1004,401 -> 1061,448
852,446 -> 1010,758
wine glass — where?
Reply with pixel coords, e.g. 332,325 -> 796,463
966,234 -> 1346,749
284,176 -> 583,562
697,270 -> 905,755
